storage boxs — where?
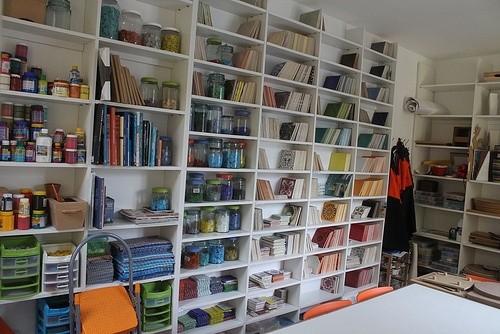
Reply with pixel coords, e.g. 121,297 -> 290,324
36,296 -> 75,334
141,281 -> 172,332
0,235 -> 40,301
41,243 -> 80,290
48,195 -> 88,230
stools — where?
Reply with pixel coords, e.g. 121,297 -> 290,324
382,249 -> 410,286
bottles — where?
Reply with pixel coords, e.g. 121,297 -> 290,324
140,77 -> 180,110
99,0 -> 181,54
205,38 -> 234,100
185,101 -> 251,203
181,206 -> 241,270
87,234 -> 110,258
150,187 -> 169,211
46,0 -> 72,30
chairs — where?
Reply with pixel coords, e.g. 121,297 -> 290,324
68,232 -> 140,334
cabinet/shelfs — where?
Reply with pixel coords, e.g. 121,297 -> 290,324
0,0 -> 399,334
404,52 -> 500,283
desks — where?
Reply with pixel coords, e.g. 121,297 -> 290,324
265,284 -> 500,334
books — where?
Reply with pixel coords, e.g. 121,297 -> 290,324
238,20 -> 261,39
220,275 -> 237,283
119,207 -> 179,225
247,31 -> 394,312
88,172 -> 106,229
197,1 -> 212,26
97,47 -> 146,105
232,50 -> 258,71
192,71 -> 205,96
224,80 -> 257,103
91,104 -> 172,167
